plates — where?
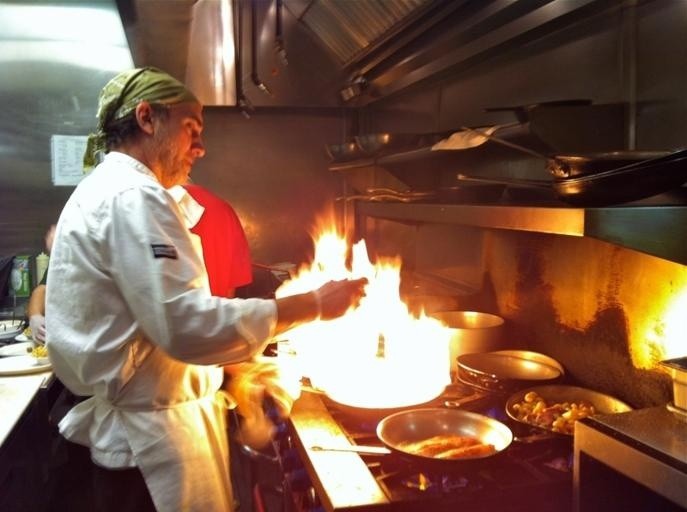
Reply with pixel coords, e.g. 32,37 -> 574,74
0,318 -> 53,377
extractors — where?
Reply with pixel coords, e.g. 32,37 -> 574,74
281,1 -> 621,109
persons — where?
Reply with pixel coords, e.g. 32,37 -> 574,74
27,224 -> 57,345
176,182 -> 253,297
43,67 -> 367,512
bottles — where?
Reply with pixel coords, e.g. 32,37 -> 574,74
36,251 -> 49,285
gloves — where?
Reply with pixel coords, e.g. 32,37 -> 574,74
29,314 -> 46,346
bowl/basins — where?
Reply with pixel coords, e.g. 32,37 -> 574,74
436,309 -> 506,355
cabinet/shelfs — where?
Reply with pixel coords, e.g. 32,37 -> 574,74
341,0 -> 687,267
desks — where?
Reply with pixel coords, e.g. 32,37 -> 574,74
0,370 -> 62,512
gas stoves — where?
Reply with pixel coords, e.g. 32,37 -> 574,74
281,373 -> 648,512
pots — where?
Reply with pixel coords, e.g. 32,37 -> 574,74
444,350 -> 564,410
308,407 -> 513,473
292,350 -> 450,418
504,383 -> 634,447
